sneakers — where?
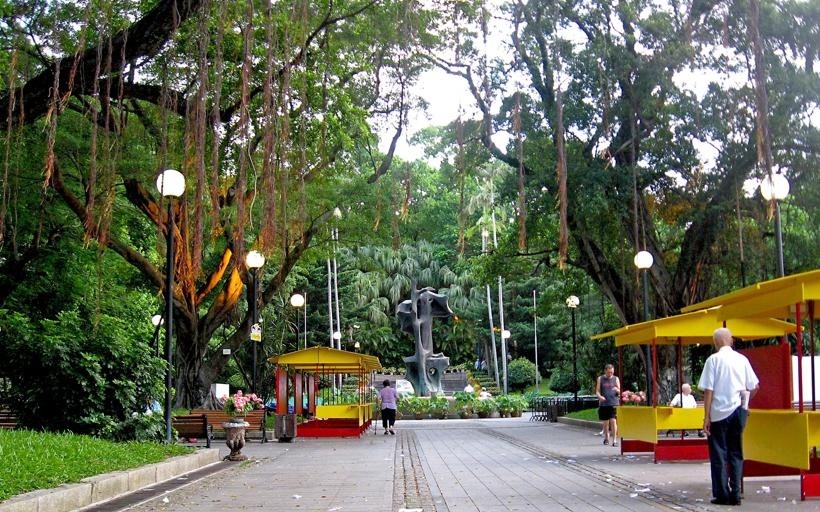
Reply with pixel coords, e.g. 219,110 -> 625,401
698,431 -> 706,437
385,431 -> 389,434
685,432 -> 689,436
389,429 -> 394,434
604,439 -> 609,445
613,443 -> 618,446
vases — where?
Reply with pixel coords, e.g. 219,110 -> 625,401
221,420 -> 250,463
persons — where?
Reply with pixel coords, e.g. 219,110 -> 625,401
670,382 -> 706,438
473,351 -> 514,370
594,363 -> 622,448
696,327 -> 760,506
354,378 -> 400,435
464,379 -> 492,398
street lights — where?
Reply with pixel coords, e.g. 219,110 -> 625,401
156,169 -> 187,444
565,295 -> 582,396
760,175 -> 792,281
246,248 -> 267,407
152,311 -> 164,356
333,333 -> 342,347
503,330 -> 512,393
633,249 -> 656,405
289,292 -> 305,352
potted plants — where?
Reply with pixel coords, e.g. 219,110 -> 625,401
372,392 -> 538,420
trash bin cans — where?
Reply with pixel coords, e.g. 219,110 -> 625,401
274,413 -> 297,443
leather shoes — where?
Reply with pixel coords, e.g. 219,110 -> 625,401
711,499 -> 740,505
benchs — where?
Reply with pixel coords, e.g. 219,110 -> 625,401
132,413 -> 214,448
665,398 -> 707,438
0,400 -> 29,429
190,408 -> 269,445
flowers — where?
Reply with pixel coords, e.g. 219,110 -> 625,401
219,388 -> 267,420
